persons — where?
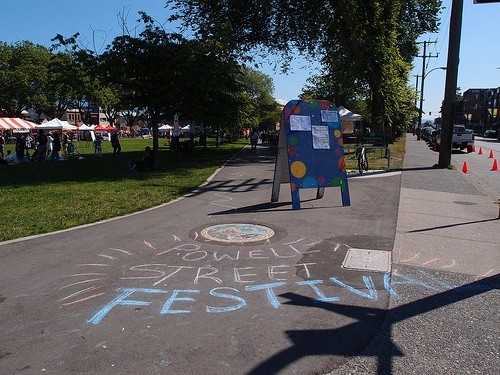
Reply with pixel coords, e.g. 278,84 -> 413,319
261,130 -> 278,145
250,127 -> 259,152
15,130 -> 61,161
0,133 -> 12,162
111,131 -> 121,153
93,136 -> 105,158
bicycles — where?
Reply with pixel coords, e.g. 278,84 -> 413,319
354,142 -> 369,175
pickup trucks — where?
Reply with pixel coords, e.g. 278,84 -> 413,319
450,124 -> 474,153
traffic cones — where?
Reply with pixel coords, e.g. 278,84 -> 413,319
478,147 -> 482,154
472,145 -> 475,152
490,159 -> 499,171
462,161 -> 469,173
488,150 -> 494,158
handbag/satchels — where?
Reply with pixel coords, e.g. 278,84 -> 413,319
56,145 -> 61,150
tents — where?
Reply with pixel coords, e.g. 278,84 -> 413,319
338,105 -> 362,122
158,124 -> 190,129
0,118 -> 114,131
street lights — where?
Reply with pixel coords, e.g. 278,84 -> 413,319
417,67 -> 446,141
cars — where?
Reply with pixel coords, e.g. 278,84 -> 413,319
416,127 -> 441,152
484,130 -> 497,138
